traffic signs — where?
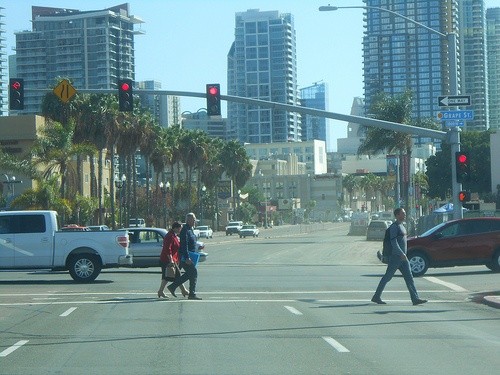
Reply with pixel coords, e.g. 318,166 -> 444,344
437,96 -> 470,106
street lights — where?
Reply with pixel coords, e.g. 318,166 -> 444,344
114,173 -> 127,229
159,181 -> 171,229
195,184 -> 207,227
237,188 -> 241,222
318,2 -> 464,220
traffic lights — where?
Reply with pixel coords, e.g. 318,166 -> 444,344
8,77 -> 24,110
454,151 -> 469,163
459,191 -> 471,202
206,84 -> 222,116
118,78 -> 134,112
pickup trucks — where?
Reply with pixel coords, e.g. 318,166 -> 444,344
0,211 -> 133,283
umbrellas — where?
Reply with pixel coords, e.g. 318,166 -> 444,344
433,203 -> 467,214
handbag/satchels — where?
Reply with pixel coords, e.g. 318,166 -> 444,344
166,263 -> 176,278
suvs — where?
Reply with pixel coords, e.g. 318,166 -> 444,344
223,220 -> 259,237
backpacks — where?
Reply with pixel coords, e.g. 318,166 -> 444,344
383,223 -> 395,256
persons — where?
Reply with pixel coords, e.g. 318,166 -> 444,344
166,213 -> 202,300
370,207 -> 427,306
157,221 -> 189,299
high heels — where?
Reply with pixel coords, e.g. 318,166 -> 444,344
182,289 -> 196,297
158,292 -> 169,298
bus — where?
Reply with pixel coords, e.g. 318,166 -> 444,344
127,219 -> 147,228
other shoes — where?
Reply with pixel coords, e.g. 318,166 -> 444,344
188,296 -> 202,300
371,298 -> 386,304
413,299 -> 427,305
167,285 -> 177,297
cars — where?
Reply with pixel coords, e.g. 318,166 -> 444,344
192,226 -> 214,240
351,212 -> 395,240
116,227 -> 208,269
376,217 -> 500,277
